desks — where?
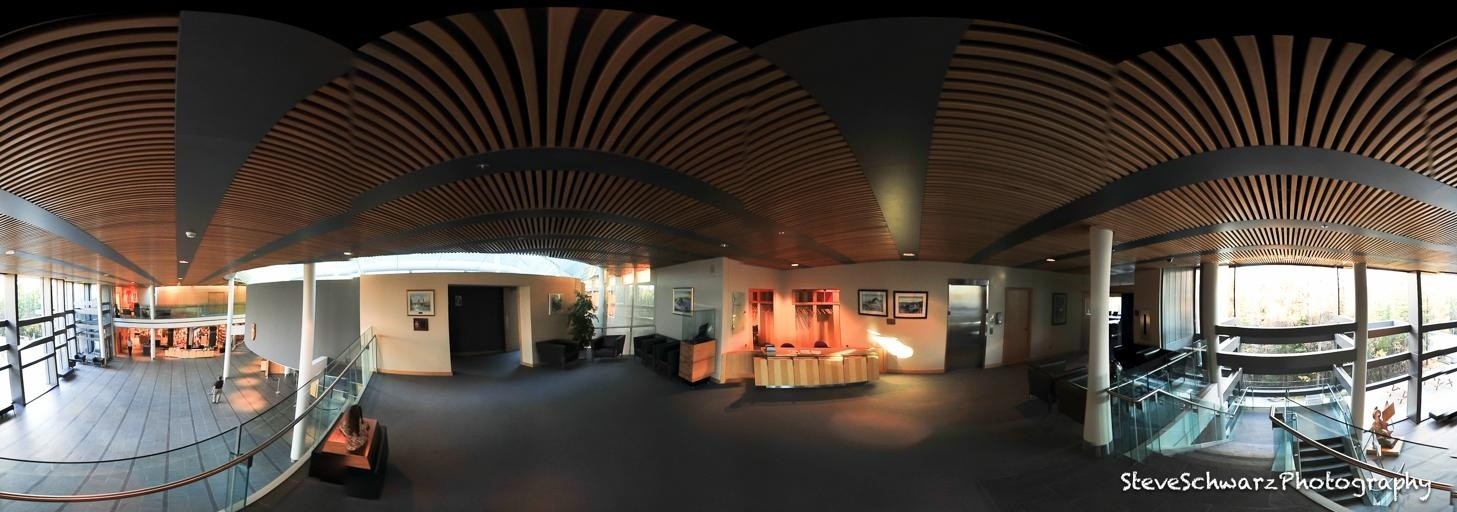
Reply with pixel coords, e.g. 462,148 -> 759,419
164,346 -> 220,357
143,344 -> 150,356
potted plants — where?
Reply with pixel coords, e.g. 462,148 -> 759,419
565,291 -> 599,360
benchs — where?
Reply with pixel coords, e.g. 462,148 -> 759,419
57,367 -> 74,378
156,309 -> 170,318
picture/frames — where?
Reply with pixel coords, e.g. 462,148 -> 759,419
858,289 -> 888,317
548,293 -> 565,316
407,289 -> 436,317
893,290 -> 928,319
672,287 -> 693,317
413,318 -> 428,330
1052,293 -> 1067,325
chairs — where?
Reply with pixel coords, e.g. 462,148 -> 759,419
1026,343 -> 1186,425
634,334 -> 680,379
68,359 -> 76,368
123,309 -> 133,318
75,354 -> 86,362
535,338 -> 577,370
93,357 -> 105,368
591,335 -> 626,360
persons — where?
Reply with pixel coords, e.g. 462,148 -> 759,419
212,376 -> 223,403
127,337 -> 132,357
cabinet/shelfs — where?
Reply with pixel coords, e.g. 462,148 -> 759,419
679,338 -> 717,386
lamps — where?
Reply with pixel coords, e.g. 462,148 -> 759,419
185,232 -> 197,239
